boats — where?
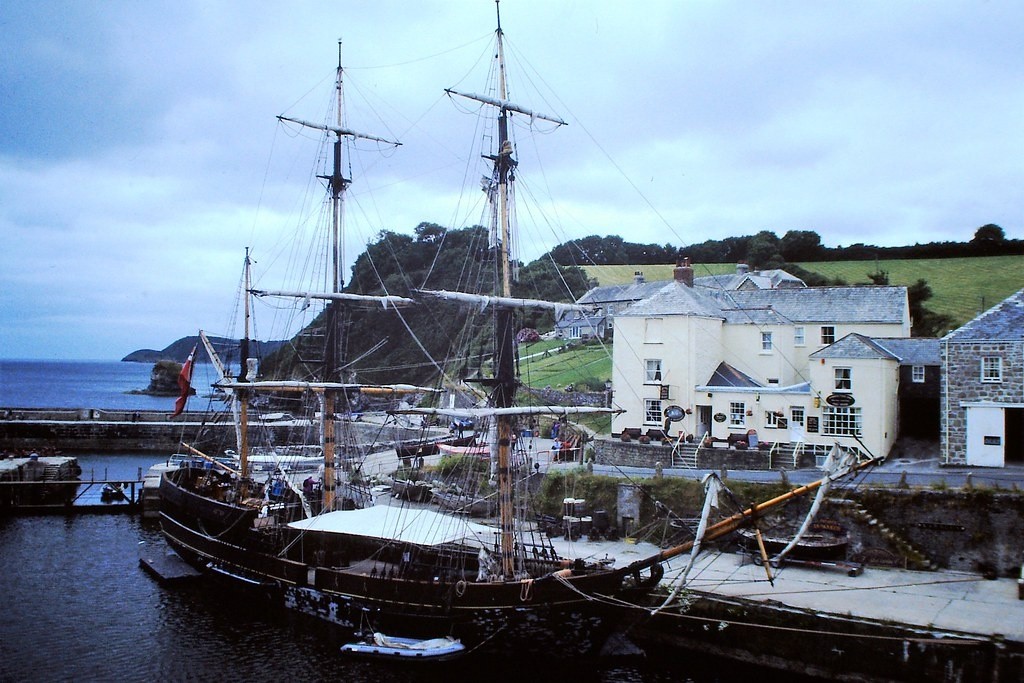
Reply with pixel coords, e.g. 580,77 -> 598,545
388,474 -> 430,504
431,438 -> 493,459
393,432 -> 482,459
100,483 -> 125,499
428,485 -> 501,516
734,527 -> 851,557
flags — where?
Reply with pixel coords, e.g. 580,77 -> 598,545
169,346 -> 194,419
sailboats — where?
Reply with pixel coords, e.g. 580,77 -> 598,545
155,0 -> 692,661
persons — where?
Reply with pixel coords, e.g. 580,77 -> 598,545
552,438 -> 562,463
510,431 -> 516,450
449,423 -> 464,439
303,477 -> 320,492
